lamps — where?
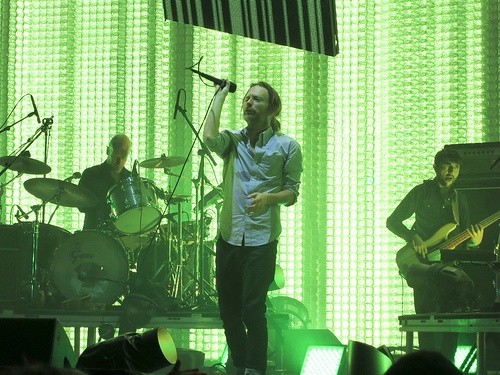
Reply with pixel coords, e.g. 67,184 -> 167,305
74,327 -> 179,375
285,329 -> 347,375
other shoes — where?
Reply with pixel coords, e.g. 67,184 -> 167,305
225,365 -> 265,375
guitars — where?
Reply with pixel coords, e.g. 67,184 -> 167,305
396,208 -> 500,288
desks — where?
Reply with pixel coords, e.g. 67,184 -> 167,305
398,312 -> 500,375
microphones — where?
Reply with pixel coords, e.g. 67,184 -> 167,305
17,205 -> 29,220
132,161 -> 136,175
189,69 -> 237,93
31,95 -> 41,123
173,90 -> 181,119
64,172 -> 82,181
78,271 -> 87,280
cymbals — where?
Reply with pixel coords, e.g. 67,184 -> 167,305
193,181 -> 224,214
0,155 -> 52,175
138,155 -> 189,169
22,177 -> 101,209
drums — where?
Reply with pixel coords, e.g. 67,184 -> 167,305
49,229 -> 130,308
104,177 -> 163,236
163,218 -> 211,242
13,222 -> 74,274
135,240 -> 217,307
163,193 -> 192,199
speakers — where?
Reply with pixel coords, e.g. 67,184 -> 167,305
0,317 -> 76,370
267,329 -> 341,375
450,177 -> 500,252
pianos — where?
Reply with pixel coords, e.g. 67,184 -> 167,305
396,310 -> 500,375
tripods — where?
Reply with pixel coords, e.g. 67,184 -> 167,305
151,109 -> 218,311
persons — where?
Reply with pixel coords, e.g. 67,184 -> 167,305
79,134 -> 157,233
201,78 -> 303,375
386,149 -> 486,364
